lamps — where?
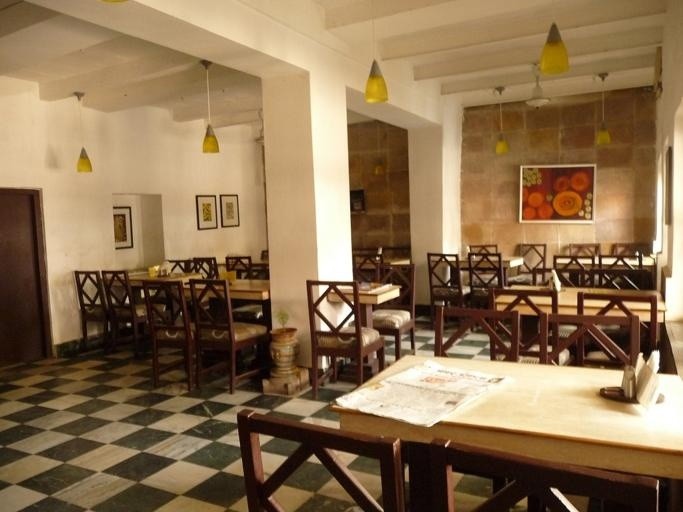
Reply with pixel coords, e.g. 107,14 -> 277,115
536,0 -> 573,78
374,115 -> 385,178
199,58 -> 221,154
594,70 -> 614,145
73,90 -> 94,174
491,84 -> 511,156
363,0 -> 392,104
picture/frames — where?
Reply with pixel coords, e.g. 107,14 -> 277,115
219,193 -> 240,228
518,163 -> 597,225
112,205 -> 135,250
195,193 -> 218,231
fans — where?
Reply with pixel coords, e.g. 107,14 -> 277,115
507,61 -> 601,109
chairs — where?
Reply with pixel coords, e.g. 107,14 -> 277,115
425,439 -> 660,512
427,242 -> 668,370
71,246 -> 415,394
237,409 -> 406,512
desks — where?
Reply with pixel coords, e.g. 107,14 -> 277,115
330,355 -> 683,511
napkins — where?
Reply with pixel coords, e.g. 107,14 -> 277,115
635,350 -> 659,376
